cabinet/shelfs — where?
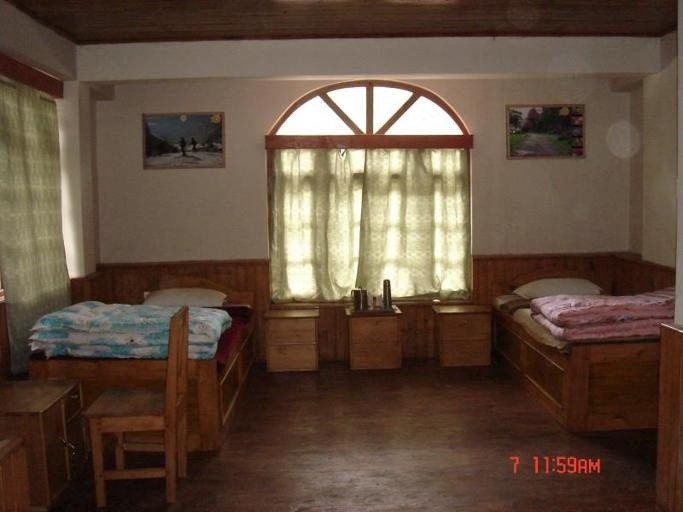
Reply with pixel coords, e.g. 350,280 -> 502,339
0,380 -> 88,507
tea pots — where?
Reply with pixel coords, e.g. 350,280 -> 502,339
351,286 -> 368,311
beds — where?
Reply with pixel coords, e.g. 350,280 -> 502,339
472,252 -> 674,435
25,259 -> 270,456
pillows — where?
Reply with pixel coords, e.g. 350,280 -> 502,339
140,287 -> 227,307
511,277 -> 604,301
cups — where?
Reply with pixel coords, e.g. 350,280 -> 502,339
372,294 -> 382,308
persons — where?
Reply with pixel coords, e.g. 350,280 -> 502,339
189,136 -> 197,150
178,136 -> 187,156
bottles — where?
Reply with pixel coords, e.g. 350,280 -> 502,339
383,279 -> 392,310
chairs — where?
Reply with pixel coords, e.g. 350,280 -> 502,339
81,306 -> 190,509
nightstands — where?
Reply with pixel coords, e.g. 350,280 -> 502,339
263,304 -> 492,374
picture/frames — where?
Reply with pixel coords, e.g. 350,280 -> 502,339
141,110 -> 225,170
504,102 -> 586,160
